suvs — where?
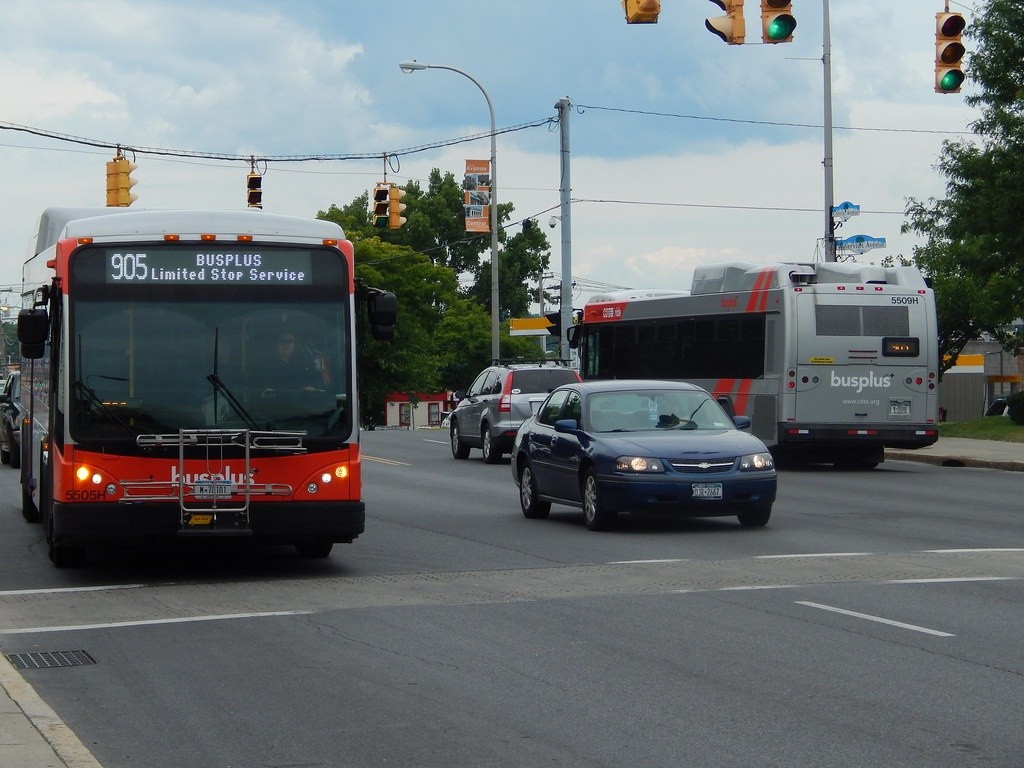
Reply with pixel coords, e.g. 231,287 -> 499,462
448,356 -> 585,465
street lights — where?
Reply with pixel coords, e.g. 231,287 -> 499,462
987,350 -> 1004,394
399,59 -> 501,367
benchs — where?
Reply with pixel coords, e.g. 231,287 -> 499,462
591,410 -> 650,432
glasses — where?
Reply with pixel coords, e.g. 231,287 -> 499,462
278,339 -> 295,343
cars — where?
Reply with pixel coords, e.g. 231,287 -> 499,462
0,370 -> 21,469
510,379 -> 778,532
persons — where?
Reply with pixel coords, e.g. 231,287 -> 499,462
248,331 -> 328,399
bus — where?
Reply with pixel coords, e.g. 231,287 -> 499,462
14,205 -> 399,568
564,258 -> 941,471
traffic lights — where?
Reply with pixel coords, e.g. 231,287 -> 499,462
372,186 -> 389,228
544,308 -> 561,338
761,0 -> 797,44
245,173 -> 263,209
387,186 -> 407,229
934,11 -> 967,94
704,0 -> 746,45
105,160 -> 139,207
